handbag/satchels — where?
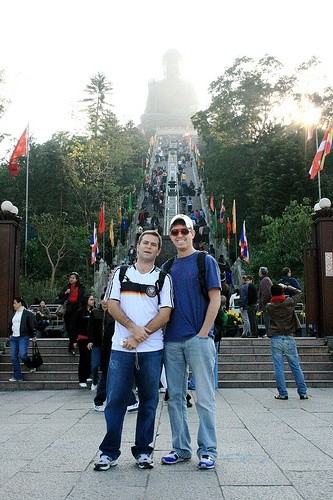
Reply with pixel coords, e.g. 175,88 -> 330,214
56,303 -> 66,318
31,341 -> 43,368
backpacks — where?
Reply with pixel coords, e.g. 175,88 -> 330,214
166,251 -> 224,343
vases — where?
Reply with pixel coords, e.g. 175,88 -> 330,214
226,325 -> 238,337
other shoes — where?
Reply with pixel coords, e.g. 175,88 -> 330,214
79,383 -> 88,387
68,348 -> 77,355
262,335 -> 272,338
30,368 -> 36,373
87,378 -> 92,381
249,335 -> 258,337
91,384 -> 97,391
9,377 -> 22,381
275,394 -> 289,399
300,395 -> 309,399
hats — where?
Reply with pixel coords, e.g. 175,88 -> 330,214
271,285 -> 284,297
170,215 -> 193,231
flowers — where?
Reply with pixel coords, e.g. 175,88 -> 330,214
226,310 -> 242,325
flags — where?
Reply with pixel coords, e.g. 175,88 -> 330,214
210,194 -> 250,268
9,129 -> 29,177
90,193 -> 135,265
309,115 -> 333,180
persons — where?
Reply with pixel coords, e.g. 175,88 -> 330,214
218,254 -> 235,338
177,165 -> 201,214
135,210 -> 163,243
240,266 -> 302,338
160,214 -> 222,469
78,292 -> 139,412
190,208 -> 210,250
141,137 -> 167,215
177,137 -> 194,168
262,281 -> 310,400
208,244 -> 215,259
35,300 -> 52,338
7,296 -> 36,381
91,229 -> 174,471
58,272 -> 86,355
128,245 -> 137,264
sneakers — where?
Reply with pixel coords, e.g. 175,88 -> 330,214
136,454 -> 155,469
127,402 -> 139,412
198,455 -> 215,469
162,450 -> 188,464
94,455 -> 117,471
93,403 -> 105,411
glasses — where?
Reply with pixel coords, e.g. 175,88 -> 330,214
170,228 -> 192,236
